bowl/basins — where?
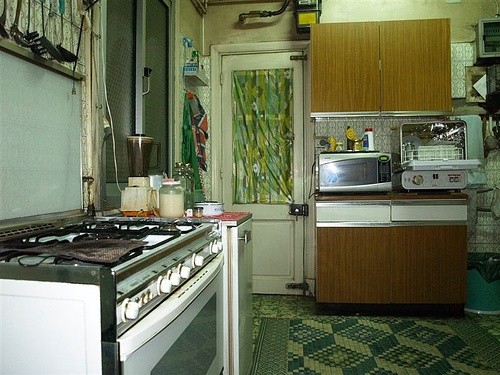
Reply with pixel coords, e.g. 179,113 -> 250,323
196,203 -> 224,216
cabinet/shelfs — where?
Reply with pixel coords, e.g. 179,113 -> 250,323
316,225 -> 467,304
310,18 -> 452,116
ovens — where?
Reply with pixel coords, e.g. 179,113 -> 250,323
0,255 -> 225,375
389,121 -> 470,194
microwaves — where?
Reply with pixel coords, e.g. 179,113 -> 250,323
317,151 -> 392,193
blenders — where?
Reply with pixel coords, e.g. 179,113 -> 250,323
120,133 -> 157,216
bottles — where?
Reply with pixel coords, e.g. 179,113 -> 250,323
363,129 -> 374,151
159,180 -> 184,218
172,161 -> 193,210
193,207 -> 203,217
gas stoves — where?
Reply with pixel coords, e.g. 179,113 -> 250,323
0,213 -> 223,341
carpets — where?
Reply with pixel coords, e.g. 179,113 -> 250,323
251,316 -> 500,375
